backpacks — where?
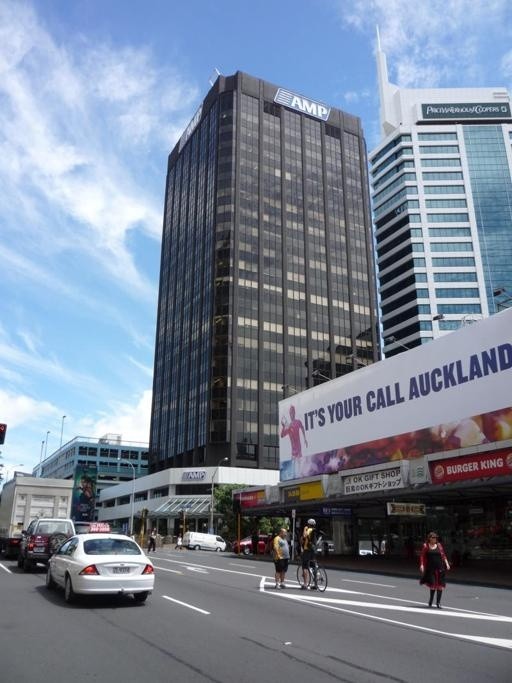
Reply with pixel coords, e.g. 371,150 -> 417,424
306,527 -> 326,557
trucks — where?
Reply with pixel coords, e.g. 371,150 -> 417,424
0,475 -> 77,553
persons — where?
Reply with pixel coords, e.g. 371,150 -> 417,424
175,534 -> 184,550
272,529 -> 292,590
251,531 -> 261,555
418,531 -> 453,609
147,527 -> 157,552
300,518 -> 320,591
76,476 -> 95,515
280,406 -> 310,460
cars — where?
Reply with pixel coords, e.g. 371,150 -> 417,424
232,530 -> 267,555
316,543 -> 334,552
44,521 -> 155,605
71,519 -> 97,533
360,548 -> 378,555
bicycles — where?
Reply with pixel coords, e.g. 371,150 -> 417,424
295,555 -> 329,591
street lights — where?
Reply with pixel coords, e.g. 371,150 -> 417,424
114,456 -> 136,534
5,463 -> 24,483
44,430 -> 50,459
39,440 -> 44,462
60,414 -> 67,448
209,456 -> 228,533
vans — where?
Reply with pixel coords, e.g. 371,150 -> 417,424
181,529 -> 227,552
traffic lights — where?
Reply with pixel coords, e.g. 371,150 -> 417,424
0,423 -> 7,444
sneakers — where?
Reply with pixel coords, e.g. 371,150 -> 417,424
310,585 -> 318,590
301,585 -> 309,591
280,583 -> 287,589
274,585 -> 281,590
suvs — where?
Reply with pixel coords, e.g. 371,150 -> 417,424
14,516 -> 75,572
